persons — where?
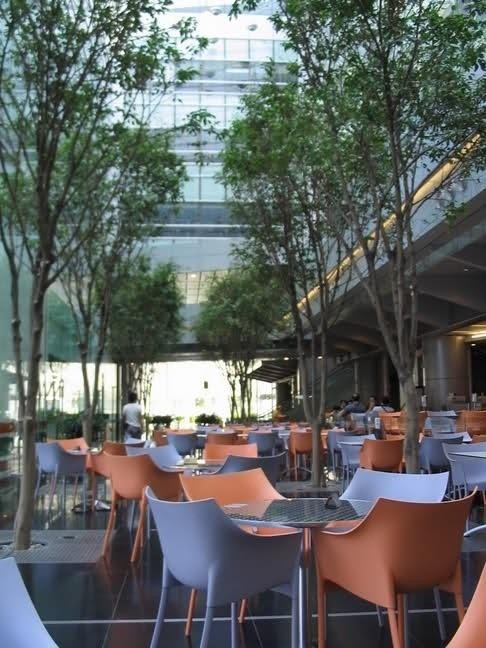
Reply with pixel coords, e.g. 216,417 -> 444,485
271,405 -> 284,422
325,394 -> 395,425
122,392 -> 144,442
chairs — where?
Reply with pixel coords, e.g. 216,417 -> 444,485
1,553 -> 61,648
99,449 -> 184,563
313,484 -> 479,648
0,396 -> 486,518
143,484 -> 305,646
336,466 -> 451,648
177,465 -> 307,637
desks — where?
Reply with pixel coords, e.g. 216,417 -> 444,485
215,497 -> 448,646
